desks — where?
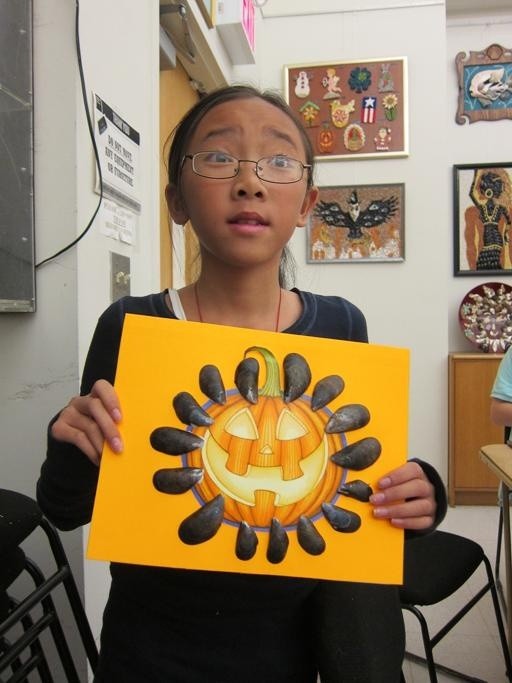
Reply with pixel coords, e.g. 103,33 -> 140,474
448,346 -> 512,627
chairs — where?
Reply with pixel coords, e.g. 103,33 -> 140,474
401,529 -> 510,681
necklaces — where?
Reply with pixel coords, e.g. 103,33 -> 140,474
194,280 -> 282,333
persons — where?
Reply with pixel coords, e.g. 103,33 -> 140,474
488,340 -> 512,509
39,87 -> 447,681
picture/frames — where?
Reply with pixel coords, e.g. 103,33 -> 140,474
302,182 -> 406,267
282,55 -> 410,164
452,160 -> 512,277
453,43 -> 510,124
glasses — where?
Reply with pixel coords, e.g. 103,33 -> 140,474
176,151 -> 316,185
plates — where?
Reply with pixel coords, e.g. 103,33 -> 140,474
458,281 -> 512,352
